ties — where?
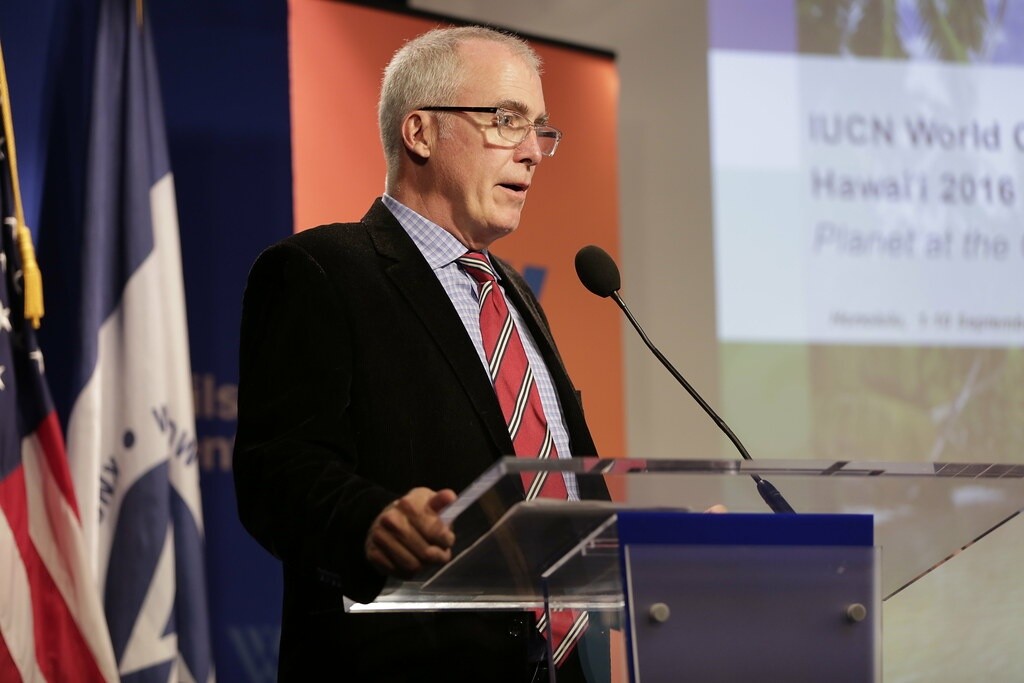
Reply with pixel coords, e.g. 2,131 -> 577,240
456,251 -> 592,667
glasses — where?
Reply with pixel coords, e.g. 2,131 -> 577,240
418,105 -> 562,157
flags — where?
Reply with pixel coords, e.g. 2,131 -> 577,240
0,47 -> 118,681
26,0 -> 219,681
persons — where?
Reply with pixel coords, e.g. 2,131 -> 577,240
227,23 -> 732,683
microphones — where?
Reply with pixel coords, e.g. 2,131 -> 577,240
574,245 -> 797,514
